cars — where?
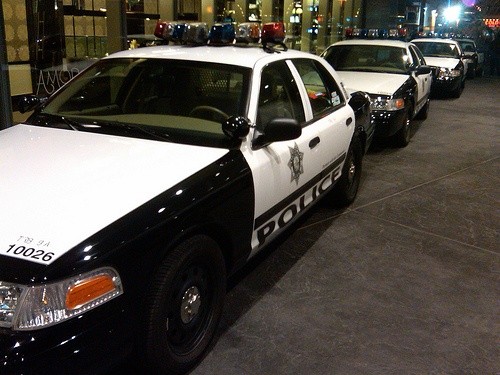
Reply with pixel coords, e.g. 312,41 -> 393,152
452,34 -> 485,78
0,19 -> 376,375
409,32 -> 468,99
301,27 -> 433,148
